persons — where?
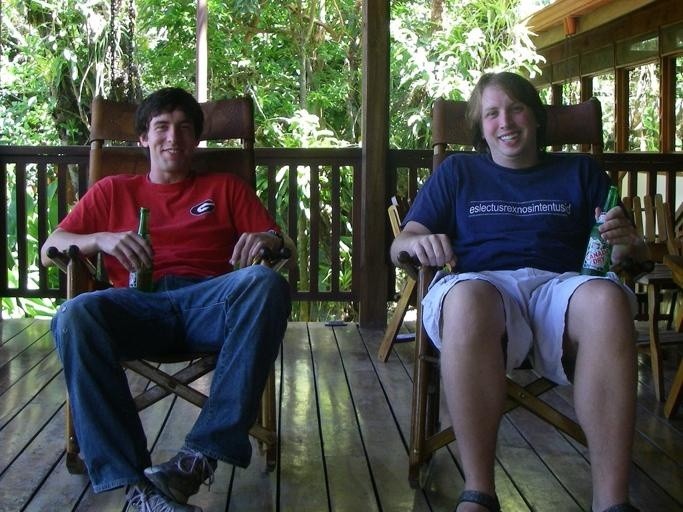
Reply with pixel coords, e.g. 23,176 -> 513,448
41,86 -> 299,511
389,71 -> 651,511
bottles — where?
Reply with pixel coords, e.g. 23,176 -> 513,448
128,205 -> 154,294
580,185 -> 620,277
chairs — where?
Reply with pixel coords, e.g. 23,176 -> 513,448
47,96 -> 290,475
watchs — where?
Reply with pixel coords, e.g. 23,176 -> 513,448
267,227 -> 286,250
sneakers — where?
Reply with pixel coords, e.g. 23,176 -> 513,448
122,480 -> 203,511
145,443 -> 214,503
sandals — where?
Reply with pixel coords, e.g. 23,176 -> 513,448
451,485 -> 502,512
